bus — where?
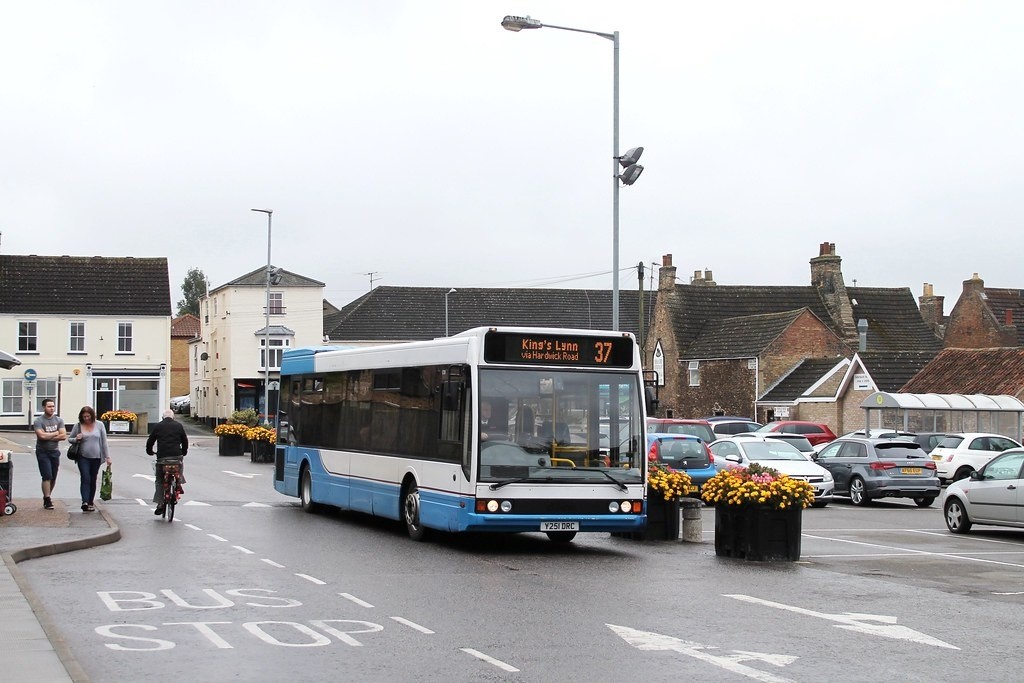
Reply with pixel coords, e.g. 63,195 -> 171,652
275,322 -> 658,545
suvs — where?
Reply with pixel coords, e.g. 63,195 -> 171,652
618,418 -> 715,443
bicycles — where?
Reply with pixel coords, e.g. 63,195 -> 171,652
153,451 -> 184,522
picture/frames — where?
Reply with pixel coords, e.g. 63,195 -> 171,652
610,497 -> 679,541
715,505 -> 802,561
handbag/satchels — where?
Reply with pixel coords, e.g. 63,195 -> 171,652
100,463 -> 112,501
67,424 -> 82,461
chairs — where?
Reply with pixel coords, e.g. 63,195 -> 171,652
541,421 -> 571,443
670,442 -> 683,453
515,406 -> 534,436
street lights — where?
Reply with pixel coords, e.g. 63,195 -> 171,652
500,15 -> 644,462
252,208 -> 274,425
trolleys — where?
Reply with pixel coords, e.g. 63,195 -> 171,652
0,485 -> 17,517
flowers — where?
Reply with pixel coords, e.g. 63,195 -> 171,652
214,407 -> 277,445
101,409 -> 138,422
700,462 -> 816,511
647,462 -> 692,501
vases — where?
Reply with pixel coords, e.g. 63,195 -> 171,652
244,439 -> 251,451
251,440 -> 275,463
108,419 -> 132,434
219,435 -> 244,456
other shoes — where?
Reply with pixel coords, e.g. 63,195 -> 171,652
81,502 -> 88,512
175,486 -> 184,495
43,495 -> 54,508
88,505 -> 95,511
154,504 -> 165,515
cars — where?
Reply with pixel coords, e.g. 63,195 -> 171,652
807,428 -> 941,507
565,410 -> 626,452
170,392 -> 191,413
617,431 -> 834,507
755,421 -> 837,446
705,417 -> 763,441
926,432 -> 1024,480
943,447 -> 1024,534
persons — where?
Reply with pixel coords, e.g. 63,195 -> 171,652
479,401 -> 513,442
33,399 -> 66,510
146,409 -> 189,515
68,406 -> 112,512
